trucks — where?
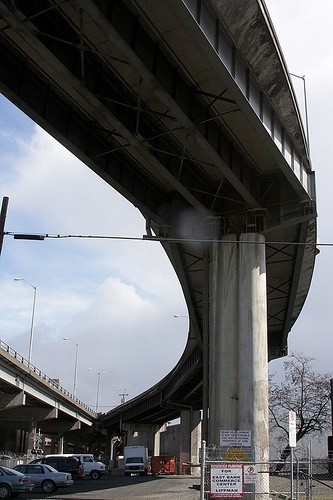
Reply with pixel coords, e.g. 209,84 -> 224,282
124,447 -> 149,476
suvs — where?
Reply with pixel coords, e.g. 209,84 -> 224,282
25,457 -> 84,477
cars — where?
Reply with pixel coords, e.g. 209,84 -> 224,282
0,455 -> 24,466
15,465 -> 73,493
0,466 -> 34,500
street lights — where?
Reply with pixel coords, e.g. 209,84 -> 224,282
88,368 -> 101,411
62,337 -> 79,397
14,277 -> 37,367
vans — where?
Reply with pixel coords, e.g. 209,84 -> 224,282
46,454 -> 109,480
